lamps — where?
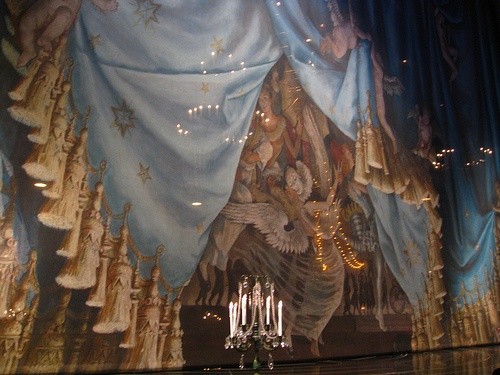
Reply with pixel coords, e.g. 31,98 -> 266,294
225,274 -> 287,375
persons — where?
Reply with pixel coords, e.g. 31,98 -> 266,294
198,83 -> 346,357
